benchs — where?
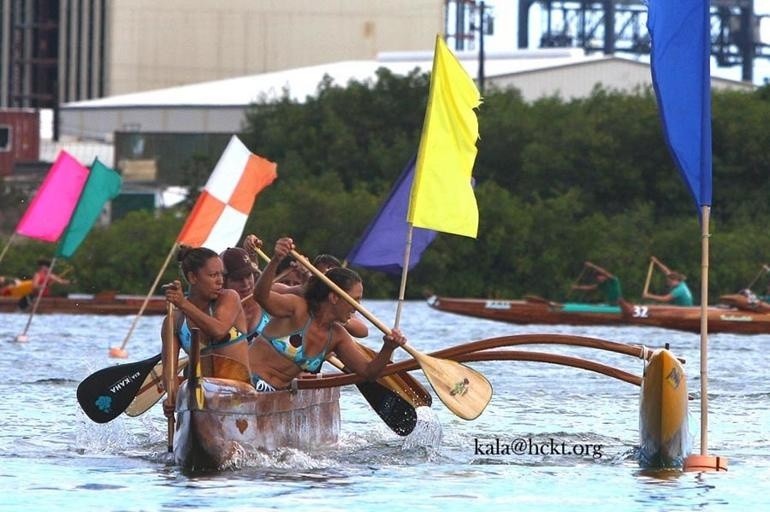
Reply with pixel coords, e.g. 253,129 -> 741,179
345,149 -> 477,273
168,133 -> 279,258
14,150 -> 89,240
53,159 -> 127,264
645,2 -> 717,210
401,32 -> 480,239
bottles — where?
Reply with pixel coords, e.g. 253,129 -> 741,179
221,246 -> 259,279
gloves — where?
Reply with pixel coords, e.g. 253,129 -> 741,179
0,275 -> 37,297
166,332 -> 689,476
0,295 -> 182,316
423,292 -> 769,335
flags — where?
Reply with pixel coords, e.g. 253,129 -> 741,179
14,150 -> 89,240
345,149 -> 477,273
168,133 -> 279,258
401,32 -> 480,239
53,159 -> 127,264
645,2 -> 717,210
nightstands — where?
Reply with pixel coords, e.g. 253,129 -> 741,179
77,264 -> 292,422
124,356 -> 190,417
18,266 -> 73,310
326,356 -> 417,436
290,245 -> 492,420
255,247 -> 432,408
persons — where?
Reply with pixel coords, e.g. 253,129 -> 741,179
643,256 -> 694,308
164,231 -> 407,401
762,263 -> 770,282
31,260 -> 71,298
569,261 -> 623,306
